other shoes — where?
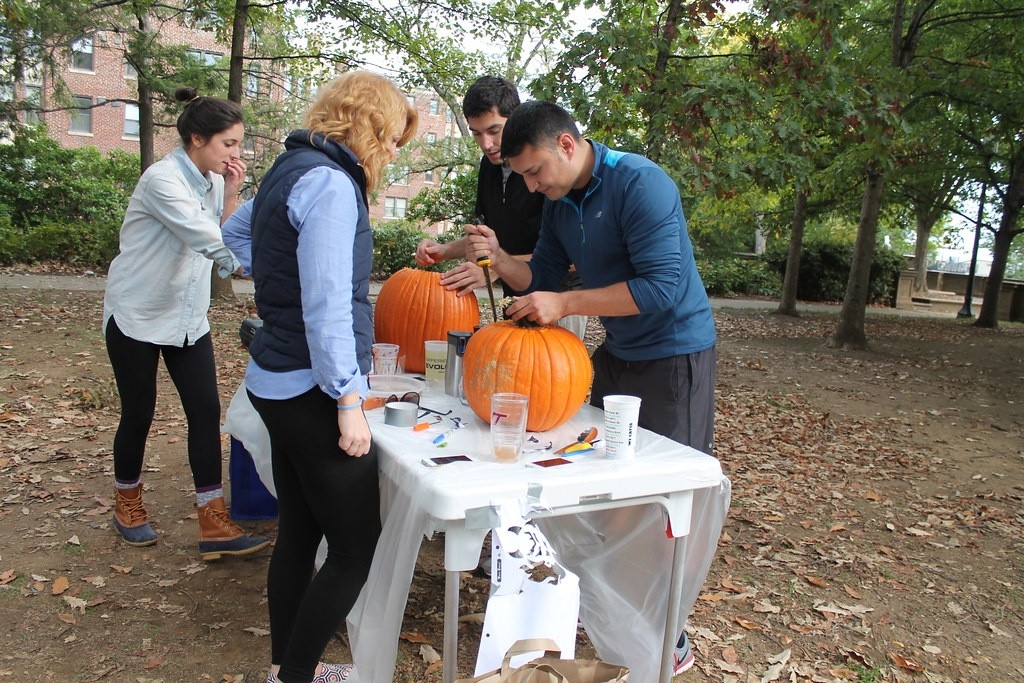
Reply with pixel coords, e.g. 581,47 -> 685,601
267,661 -> 360,683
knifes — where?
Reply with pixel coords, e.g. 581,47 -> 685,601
473,215 -> 497,323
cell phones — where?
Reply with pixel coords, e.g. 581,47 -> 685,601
412,376 -> 425,382
422,454 -> 478,469
524,457 -> 576,470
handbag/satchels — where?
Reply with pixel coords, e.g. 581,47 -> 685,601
455,638 -> 631,682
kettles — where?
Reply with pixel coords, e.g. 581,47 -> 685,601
445,330 -> 473,397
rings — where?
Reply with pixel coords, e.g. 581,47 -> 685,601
243,170 -> 247,173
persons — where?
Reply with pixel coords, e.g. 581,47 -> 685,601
103,88 -> 271,561
465,100 -> 715,683
415,77 -> 587,343
221,69 -> 418,683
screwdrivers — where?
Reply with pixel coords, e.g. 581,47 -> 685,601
413,421 -> 441,431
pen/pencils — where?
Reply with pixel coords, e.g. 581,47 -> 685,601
432,428 -> 457,444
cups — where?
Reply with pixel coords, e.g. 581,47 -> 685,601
425,340 -> 448,397
490,394 -> 529,464
372,343 -> 400,375
603,395 -> 642,462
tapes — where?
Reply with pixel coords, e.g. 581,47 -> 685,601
384,401 -> 418,427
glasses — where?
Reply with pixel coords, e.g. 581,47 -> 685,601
385,392 -> 452,420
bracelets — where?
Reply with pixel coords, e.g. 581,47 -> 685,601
337,399 -> 362,409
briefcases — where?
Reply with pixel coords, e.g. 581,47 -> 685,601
239,316 -> 266,346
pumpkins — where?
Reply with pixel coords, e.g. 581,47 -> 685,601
463,296 -> 592,432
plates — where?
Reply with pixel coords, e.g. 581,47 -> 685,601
367,375 -> 424,399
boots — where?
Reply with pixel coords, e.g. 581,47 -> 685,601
111,482 -> 159,547
193,496 -> 271,562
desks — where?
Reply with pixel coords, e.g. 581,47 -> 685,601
222,359 -> 732,683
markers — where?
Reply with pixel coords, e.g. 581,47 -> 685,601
450,417 -> 465,429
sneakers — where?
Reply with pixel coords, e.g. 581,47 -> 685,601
672,631 -> 695,677
